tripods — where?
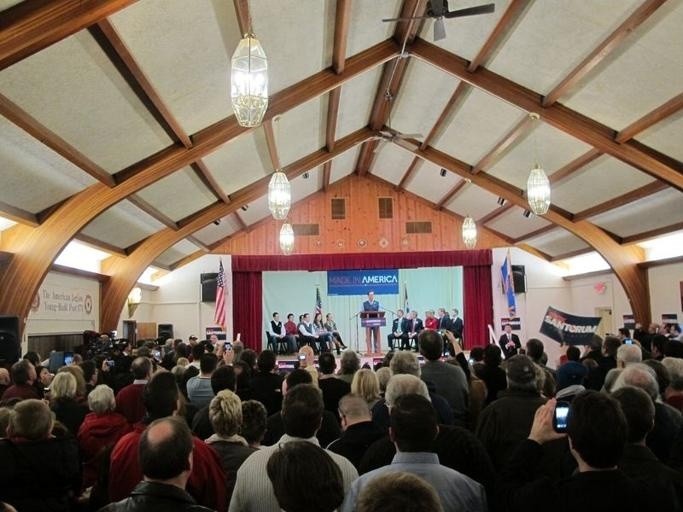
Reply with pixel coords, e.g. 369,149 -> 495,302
355,316 -> 363,356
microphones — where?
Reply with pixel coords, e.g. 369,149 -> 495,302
376,304 -> 385,310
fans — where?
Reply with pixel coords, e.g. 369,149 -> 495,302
381,1 -> 497,42
354,89 -> 425,155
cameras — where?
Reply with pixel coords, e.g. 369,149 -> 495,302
624,339 -> 632,344
436,328 -> 447,336
224,342 -> 234,352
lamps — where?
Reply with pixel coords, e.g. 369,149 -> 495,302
459,175 -> 478,251
526,111 -> 552,217
229,0 -> 296,257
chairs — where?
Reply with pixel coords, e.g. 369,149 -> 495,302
266,330 -> 337,357
392,322 -> 465,351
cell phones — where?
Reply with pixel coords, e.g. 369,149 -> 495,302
553,400 -> 571,432
297,353 -> 306,360
107,360 -> 114,366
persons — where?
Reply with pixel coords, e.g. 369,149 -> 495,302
268,311 -> 348,356
1,322 -> 682,512
363,290 -> 380,356
387,308 -> 464,354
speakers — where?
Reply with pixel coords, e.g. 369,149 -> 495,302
502,265 -> 525,294
200,273 -> 218,302
158,324 -> 174,344
0,315 -> 20,366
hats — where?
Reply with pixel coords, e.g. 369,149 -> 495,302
189,335 -> 197,340
506,355 -> 535,375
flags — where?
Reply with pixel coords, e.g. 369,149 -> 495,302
211,259 -> 226,330
403,283 -> 409,313
500,246 -> 515,320
313,287 -> 322,314
